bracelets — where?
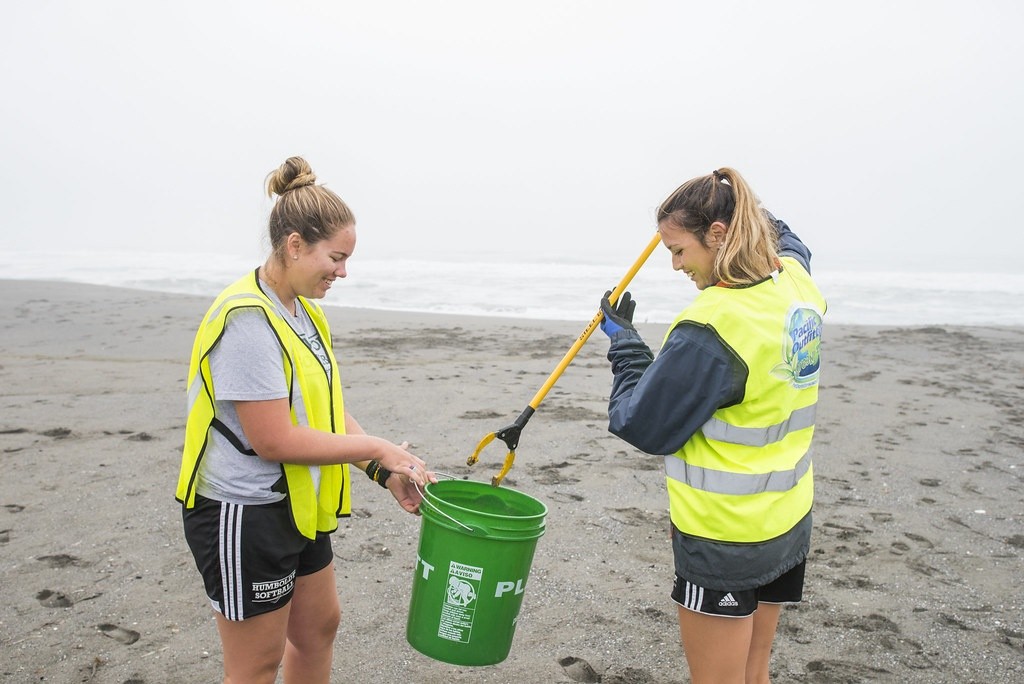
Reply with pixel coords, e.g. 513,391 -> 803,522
365,460 -> 392,489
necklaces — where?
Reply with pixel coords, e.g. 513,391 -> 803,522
264,269 -> 299,317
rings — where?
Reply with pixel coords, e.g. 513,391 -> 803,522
409,464 -> 416,471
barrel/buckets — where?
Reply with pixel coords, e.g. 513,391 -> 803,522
406,471 -> 549,666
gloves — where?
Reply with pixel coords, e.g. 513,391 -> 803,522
599,288 -> 637,338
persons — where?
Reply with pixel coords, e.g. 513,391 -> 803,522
598,165 -> 828,684
176,154 -> 437,683
